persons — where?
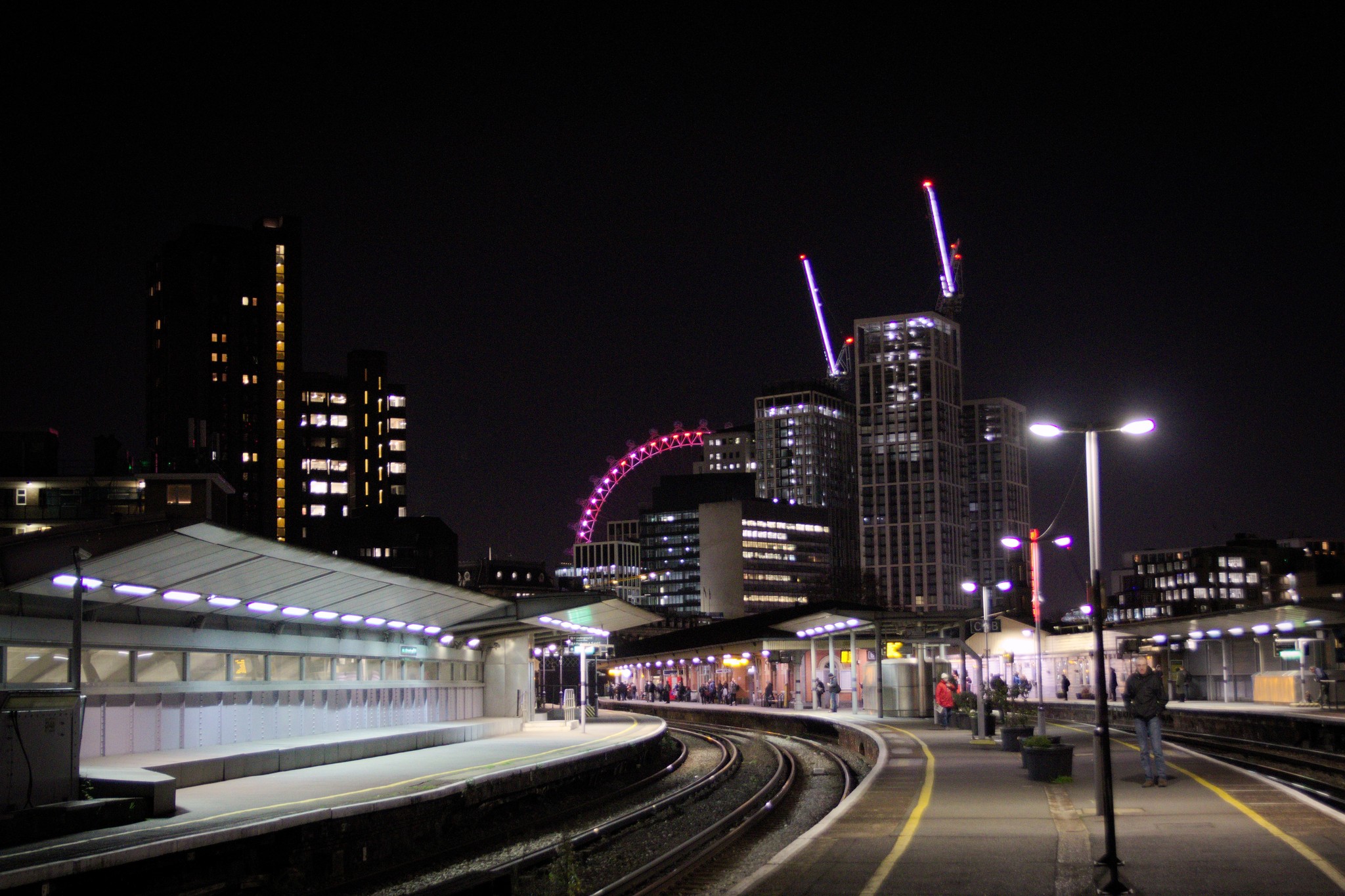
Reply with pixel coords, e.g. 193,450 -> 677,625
1108,667 -> 1118,702
1174,667 -> 1185,703
1011,672 -> 1021,699
1309,666 -> 1331,706
728,678 -> 738,706
1060,674 -> 1070,701
1152,665 -> 1165,703
815,678 -> 825,709
1018,674 -> 1028,699
859,681 -> 865,710
1024,680 -> 1032,698
602,677 -> 729,705
949,669 -> 973,698
763,680 -> 775,708
988,672 -> 1008,695
574,683 -> 581,706
826,672 -> 838,713
1179,666 -> 1193,701
1122,656 -> 1170,787
935,673 -> 956,730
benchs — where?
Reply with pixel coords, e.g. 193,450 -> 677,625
761,691 -> 786,708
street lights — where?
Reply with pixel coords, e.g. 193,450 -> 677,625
958,580 -> 1011,714
1029,418 -> 1158,895
1000,529 -> 1071,733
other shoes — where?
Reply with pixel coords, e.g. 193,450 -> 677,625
1110,699 -> 1115,701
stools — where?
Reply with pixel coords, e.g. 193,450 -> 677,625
76,716 -> 519,817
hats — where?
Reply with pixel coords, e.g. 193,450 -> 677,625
828,673 -> 834,677
941,673 -> 948,678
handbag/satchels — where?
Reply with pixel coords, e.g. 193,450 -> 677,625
834,685 -> 841,693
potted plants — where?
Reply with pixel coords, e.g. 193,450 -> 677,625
1023,736 -> 1075,780
978,674 -> 1038,752
948,690 -> 995,736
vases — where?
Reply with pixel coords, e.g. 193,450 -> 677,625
1017,735 -> 1061,769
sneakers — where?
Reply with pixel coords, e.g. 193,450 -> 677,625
1142,780 -> 1154,787
817,707 -> 821,709
1158,779 -> 1167,787
828,710 -> 837,712
